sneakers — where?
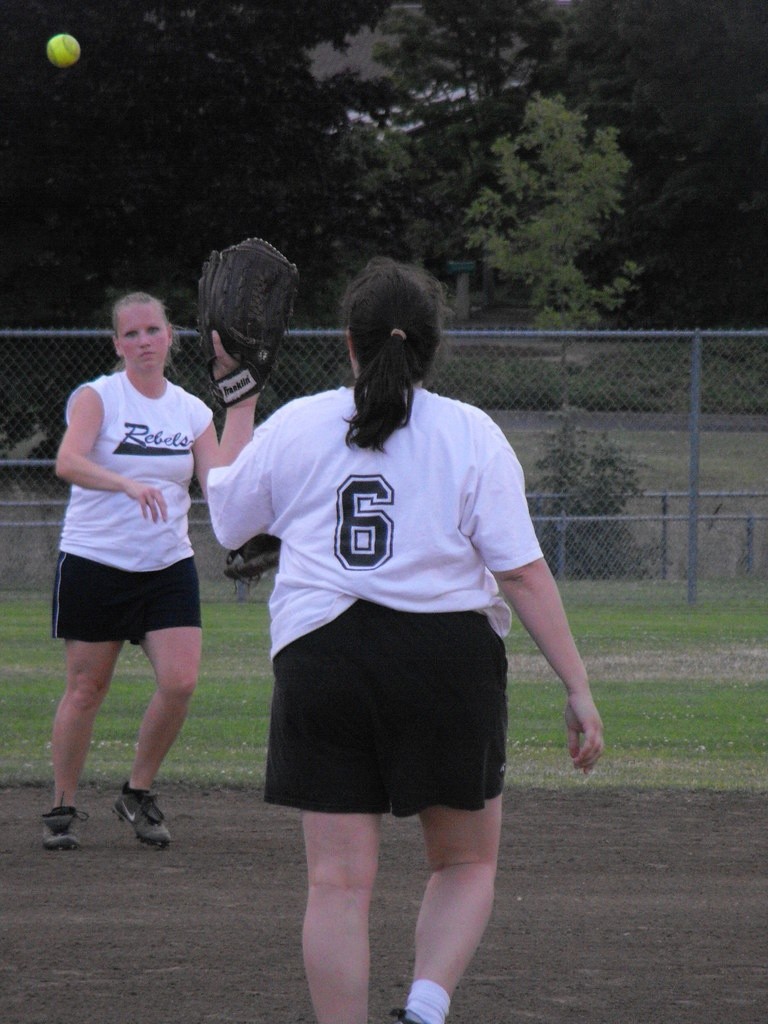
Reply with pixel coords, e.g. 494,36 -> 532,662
112,782 -> 171,848
43,792 -> 89,850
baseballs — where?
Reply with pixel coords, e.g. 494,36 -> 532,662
46,33 -> 81,69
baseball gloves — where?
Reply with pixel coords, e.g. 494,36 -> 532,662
195,236 -> 301,409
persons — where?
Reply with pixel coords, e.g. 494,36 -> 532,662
39,290 -> 282,848
210,260 -> 607,1024
